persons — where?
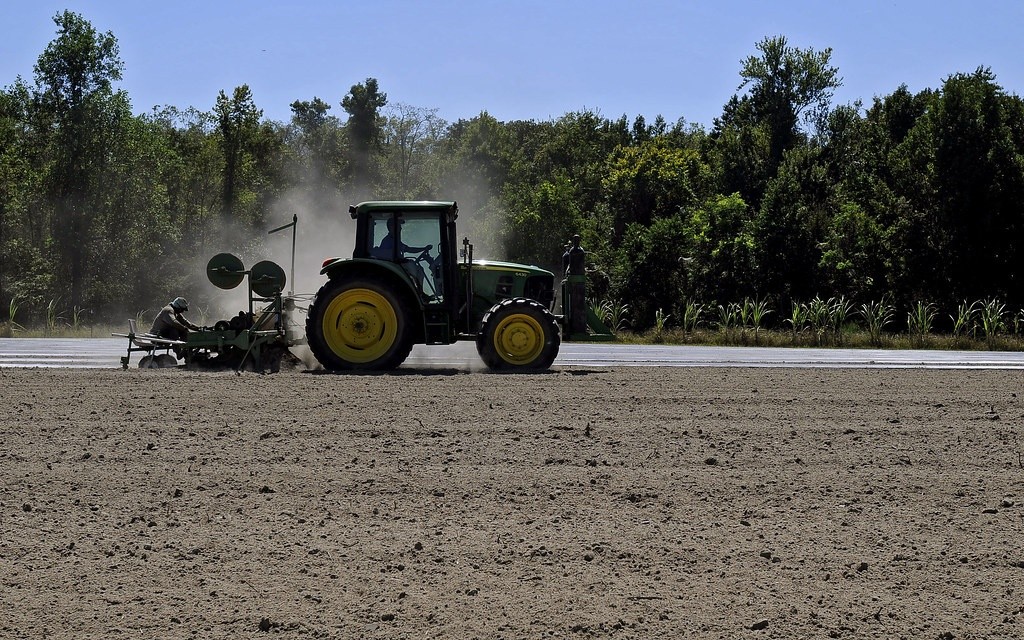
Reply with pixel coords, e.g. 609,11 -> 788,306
378,219 -> 435,302
149,297 -> 199,360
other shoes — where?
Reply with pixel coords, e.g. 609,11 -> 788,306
421,292 -> 434,302
177,350 -> 186,359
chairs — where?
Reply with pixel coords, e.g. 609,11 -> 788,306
128,318 -> 157,347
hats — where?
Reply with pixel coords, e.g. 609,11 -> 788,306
387,217 -> 405,228
172,296 -> 189,312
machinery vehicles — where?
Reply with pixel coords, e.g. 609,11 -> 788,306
111,201 -> 571,375
